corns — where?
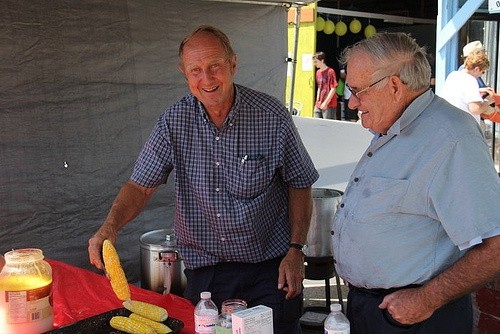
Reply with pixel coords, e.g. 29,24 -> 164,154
103,240 -> 131,300
109,300 -> 172,334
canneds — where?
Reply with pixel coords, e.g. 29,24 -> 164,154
0,248 -> 54,334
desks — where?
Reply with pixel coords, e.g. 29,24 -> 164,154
0,257 -> 196,334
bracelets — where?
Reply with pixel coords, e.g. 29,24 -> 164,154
483,98 -> 490,102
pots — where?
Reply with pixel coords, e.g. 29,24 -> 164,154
139,228 -> 190,296
301,188 -> 344,257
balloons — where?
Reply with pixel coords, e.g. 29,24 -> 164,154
316,17 -> 326,31
335,21 -> 347,36
364,25 -> 376,38
323,20 -> 335,35
349,19 -> 362,33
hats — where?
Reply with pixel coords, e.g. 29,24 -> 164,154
463,40 -> 485,57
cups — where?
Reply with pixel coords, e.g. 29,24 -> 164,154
193,291 -> 219,334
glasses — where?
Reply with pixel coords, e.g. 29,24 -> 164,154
345,74 -> 407,102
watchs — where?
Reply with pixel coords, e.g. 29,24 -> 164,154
289,242 -> 310,257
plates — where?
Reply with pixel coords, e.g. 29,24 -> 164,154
42,308 -> 184,334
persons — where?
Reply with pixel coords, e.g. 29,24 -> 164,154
312,51 -> 337,120
335,69 -> 359,123
88,26 -> 322,334
439,48 -> 495,125
329,32 -> 500,334
457,41 -> 495,138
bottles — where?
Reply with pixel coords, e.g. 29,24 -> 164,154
215,298 -> 247,334
324,304 -> 350,334
